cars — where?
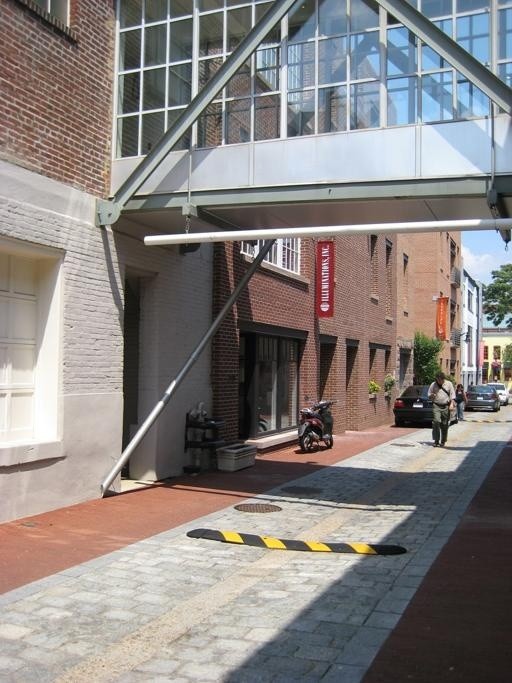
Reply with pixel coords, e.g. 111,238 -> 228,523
393,385 -> 459,428
464,383 -> 509,412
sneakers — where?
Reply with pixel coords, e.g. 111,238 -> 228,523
432,440 -> 446,447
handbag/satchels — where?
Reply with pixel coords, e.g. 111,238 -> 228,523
449,401 -> 458,422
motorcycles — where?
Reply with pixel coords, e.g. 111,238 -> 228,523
256,396 -> 268,432
298,398 -> 337,451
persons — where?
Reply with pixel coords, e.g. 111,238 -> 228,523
427,371 -> 455,447
467,381 -> 474,389
455,383 -> 467,421
